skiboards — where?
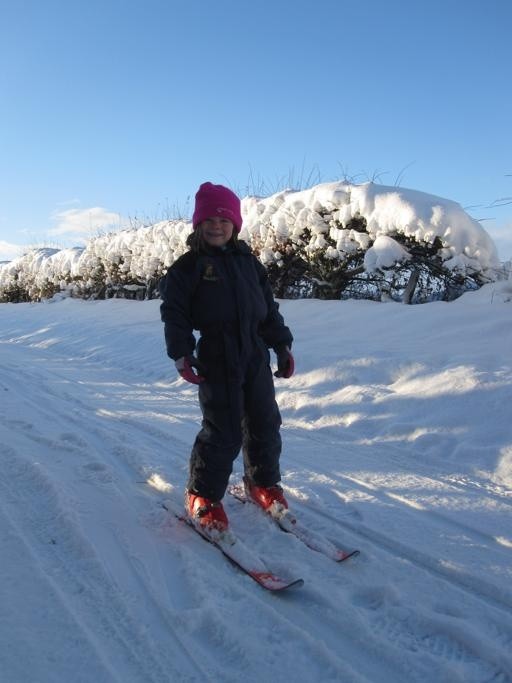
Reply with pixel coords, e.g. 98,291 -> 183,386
134,482 -> 361,593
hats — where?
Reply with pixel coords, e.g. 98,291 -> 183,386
192,182 -> 243,232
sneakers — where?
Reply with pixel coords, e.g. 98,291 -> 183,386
242,478 -> 287,513
184,491 -> 229,536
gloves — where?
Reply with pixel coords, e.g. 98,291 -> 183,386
274,346 -> 295,379
175,355 -> 208,384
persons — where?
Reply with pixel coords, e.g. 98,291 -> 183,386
158,178 -> 297,542
